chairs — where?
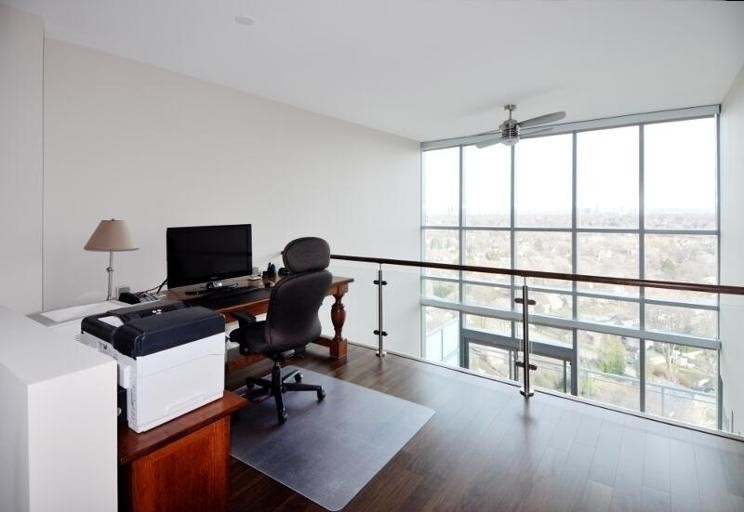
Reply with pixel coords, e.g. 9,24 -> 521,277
234,235 -> 333,427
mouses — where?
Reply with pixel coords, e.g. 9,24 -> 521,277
264,280 -> 276,288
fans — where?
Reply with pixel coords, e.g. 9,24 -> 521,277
421,104 -> 567,151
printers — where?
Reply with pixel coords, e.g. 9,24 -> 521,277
80,299 -> 226,434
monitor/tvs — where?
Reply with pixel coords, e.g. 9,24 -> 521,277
166,224 -> 253,295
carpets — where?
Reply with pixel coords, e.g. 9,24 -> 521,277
226,365 -> 437,512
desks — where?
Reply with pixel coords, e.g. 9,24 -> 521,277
148,269 -> 355,366
118,387 -> 252,511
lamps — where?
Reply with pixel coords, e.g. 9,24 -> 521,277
83,218 -> 140,299
